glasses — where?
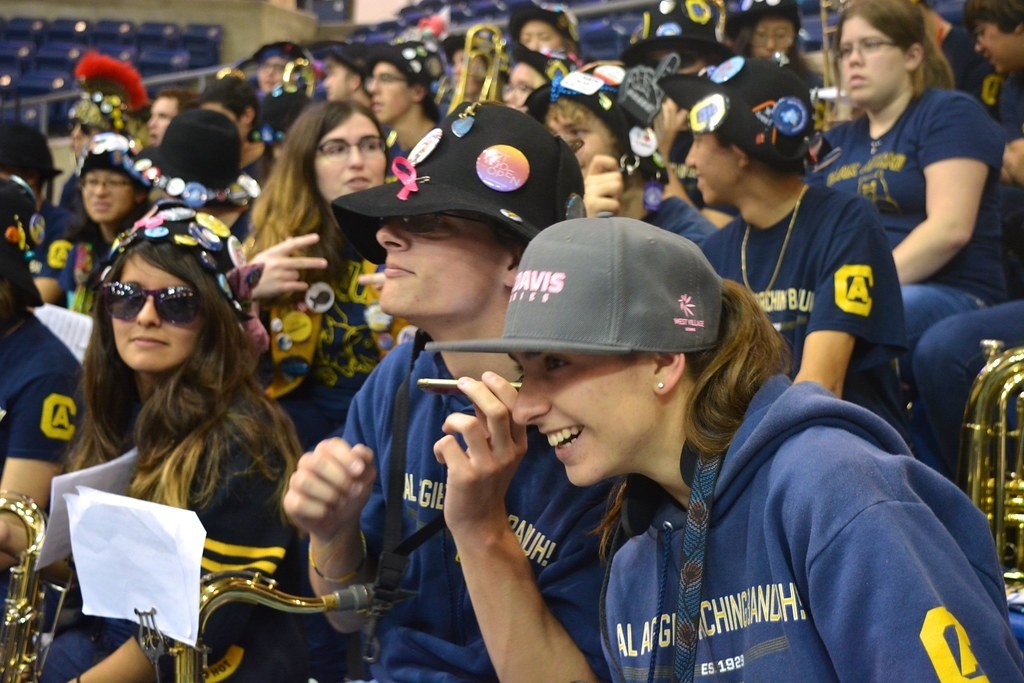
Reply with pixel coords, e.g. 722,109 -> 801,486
313,136 -> 385,158
837,39 -> 897,58
501,82 -> 535,98
69,119 -> 91,135
378,213 -> 499,235
76,176 -> 132,190
101,282 -> 205,324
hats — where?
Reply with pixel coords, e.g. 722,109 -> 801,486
0,120 -> 63,179
330,100 -> 587,265
74,132 -> 151,191
68,52 -> 150,133
423,212 -> 724,353
93,201 -> 253,321
738,0 -> 800,31
524,60 -> 643,166
619,0 -> 736,65
135,109 -> 261,206
0,176 -> 45,308
238,1 -> 583,87
657,57 -> 812,165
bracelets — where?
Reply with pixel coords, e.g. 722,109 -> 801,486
309,526 -> 366,583
76,673 -> 81,683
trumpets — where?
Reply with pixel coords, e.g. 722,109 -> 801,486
445,20 -> 509,119
282,56 -> 318,98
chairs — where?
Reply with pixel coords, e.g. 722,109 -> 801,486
0,16 -> 224,137
294,0 -> 968,106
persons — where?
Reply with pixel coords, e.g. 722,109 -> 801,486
432,210 -> 1024,683
822,1 -> 1008,478
959,1 -> 1024,188
1,0 -> 835,683
658,55 -> 916,458
914,297 -> 1024,492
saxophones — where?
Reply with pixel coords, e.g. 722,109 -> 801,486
132,569 -> 376,683
0,490 -> 76,683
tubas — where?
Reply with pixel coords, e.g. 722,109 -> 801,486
956,336 -> 1023,603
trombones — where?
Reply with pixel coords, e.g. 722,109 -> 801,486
817,1 -> 846,131
